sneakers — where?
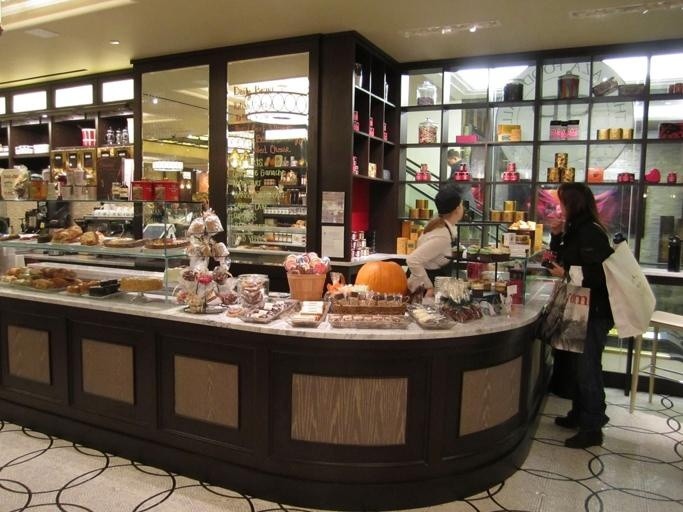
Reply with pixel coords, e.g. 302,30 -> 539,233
556,416 -> 601,448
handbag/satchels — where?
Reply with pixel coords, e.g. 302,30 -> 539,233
541,283 -> 591,353
602,241 -> 656,339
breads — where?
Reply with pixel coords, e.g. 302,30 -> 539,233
51,225 -> 98,245
234,272 -> 293,322
0,267 -> 116,294
179,217 -> 237,315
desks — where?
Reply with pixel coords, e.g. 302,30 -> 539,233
625,268 -> 682,397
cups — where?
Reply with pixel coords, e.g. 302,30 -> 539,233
155,187 -> 165,201
93,204 -> 134,217
15,255 -> 26,267
133,187 -> 143,202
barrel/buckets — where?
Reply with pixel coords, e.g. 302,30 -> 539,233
287,269 -> 326,301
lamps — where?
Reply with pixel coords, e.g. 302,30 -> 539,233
229,149 -> 251,170
244,91 -> 308,126
153,162 -> 184,172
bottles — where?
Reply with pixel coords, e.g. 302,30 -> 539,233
419,115 -> 439,143
417,80 -> 437,107
111,176 -> 128,202
105,128 -> 129,146
550,119 -> 579,141
264,229 -> 292,243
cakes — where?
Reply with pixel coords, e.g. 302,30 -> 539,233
146,239 -> 191,248
453,244 -> 510,261
103,240 -> 148,248
332,294 -> 409,316
120,276 -> 163,291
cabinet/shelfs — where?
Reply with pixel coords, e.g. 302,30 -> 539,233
0,68 -> 135,237
397,37 -> 683,361
320,30 -> 399,292
254,138 -> 308,217
0,199 -> 205,312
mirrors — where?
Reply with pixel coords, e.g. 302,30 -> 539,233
140,65 -> 210,214
224,52 -> 310,254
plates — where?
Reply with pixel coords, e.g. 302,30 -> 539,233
268,292 -> 290,298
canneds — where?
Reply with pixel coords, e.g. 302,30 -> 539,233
352,156 -> 358,166
629,174 -> 634,182
462,173 -> 469,180
460,163 -> 465,172
424,173 -> 431,180
560,120 -> 567,139
420,163 -> 428,172
667,173 -> 676,183
503,172 -> 511,181
609,128 -> 622,140
409,209 -> 420,218
502,211 -> 515,222
510,173 -> 518,181
416,172 -> 424,180
622,174 -> 630,182
383,123 -> 387,132
419,209 -> 433,219
370,117 -> 373,126
618,173 -> 623,182
546,168 -> 561,183
550,120 -> 562,140
621,129 -> 634,139
566,120 -> 580,139
507,163 -> 515,172
490,211 -> 502,222
555,153 -> 568,169
560,168 -> 574,183
514,211 -> 527,221
598,128 -> 610,140
82,128 -> 95,146
106,129 -> 129,145
455,172 -> 462,180
354,111 -> 359,120
668,84 -> 674,94
353,166 -> 359,174
351,230 -> 370,257
384,133 -> 387,140
354,122 -> 359,129
369,127 -> 375,136
415,199 -> 428,209
674,83 -> 683,94
503,201 -> 517,211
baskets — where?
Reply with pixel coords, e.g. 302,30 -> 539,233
333,300 -> 405,315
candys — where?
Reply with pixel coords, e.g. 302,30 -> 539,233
284,253 -> 332,274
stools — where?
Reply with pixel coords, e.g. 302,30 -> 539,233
630,310 -> 683,416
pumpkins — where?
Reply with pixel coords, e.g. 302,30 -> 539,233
354,262 -> 408,294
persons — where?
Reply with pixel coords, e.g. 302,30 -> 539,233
406,189 -> 463,297
447,149 -> 465,178
547,182 -> 614,448
